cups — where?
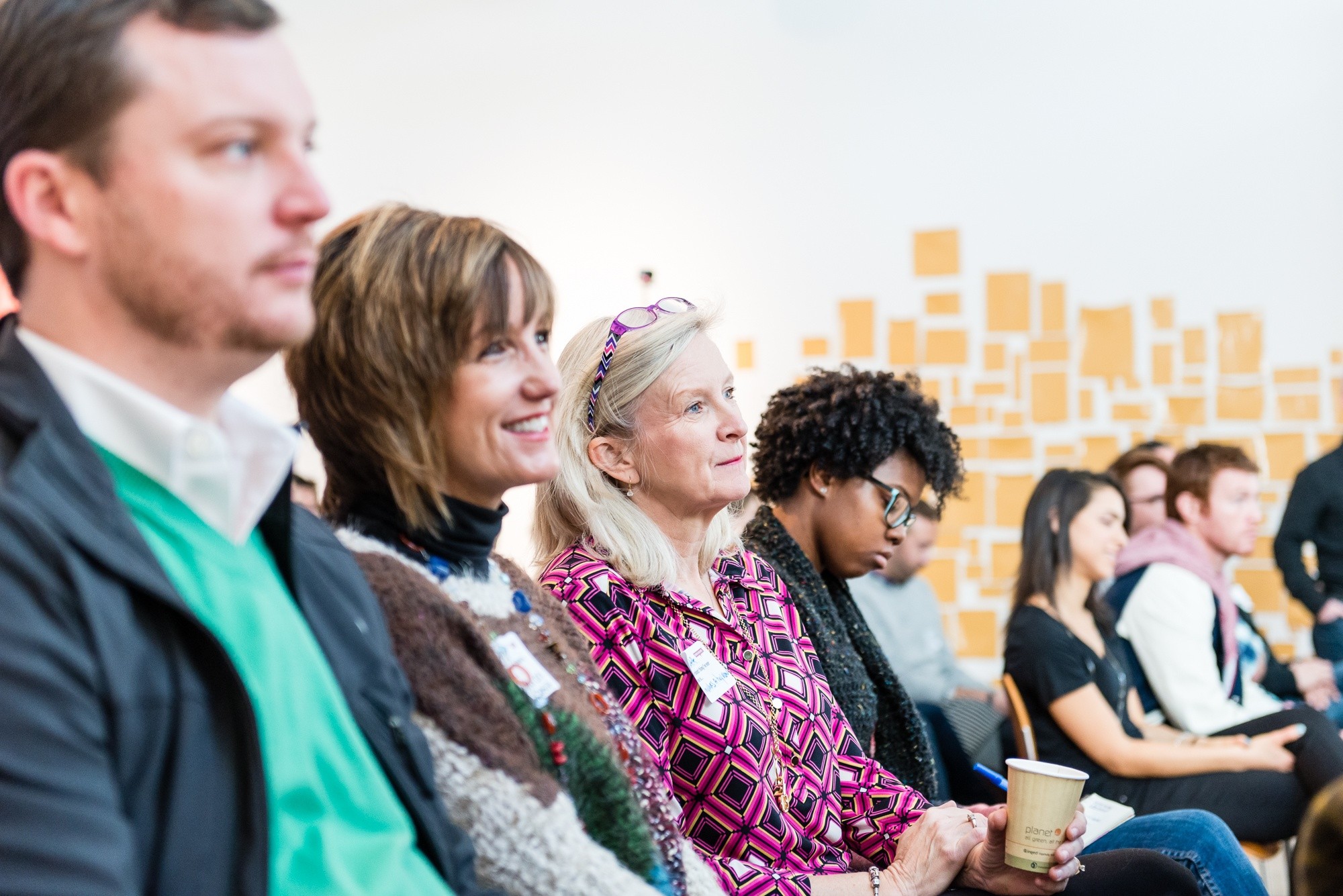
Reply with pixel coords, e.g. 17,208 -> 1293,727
1001,756 -> 1089,874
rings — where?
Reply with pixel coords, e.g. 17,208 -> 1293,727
967,813 -> 977,828
1074,857 -> 1086,876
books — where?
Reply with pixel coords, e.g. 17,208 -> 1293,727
1078,792 -> 1136,849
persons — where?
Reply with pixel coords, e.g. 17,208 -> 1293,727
1101,453 -> 1173,540
738,362 -> 1273,896
1274,445 -> 1343,670
530,305 -> 1201,896
0,1 -> 491,895
1002,466 -> 1342,843
1128,442 -> 1175,464
844,499 -> 1016,776
1098,443 -> 1284,739
274,204 -> 726,895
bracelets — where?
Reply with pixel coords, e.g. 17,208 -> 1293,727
987,693 -> 993,706
868,866 -> 880,896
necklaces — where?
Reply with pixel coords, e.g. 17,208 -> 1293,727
352,511 -> 638,840
654,582 -> 790,813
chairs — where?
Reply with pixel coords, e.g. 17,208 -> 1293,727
1002,670 -> 1290,893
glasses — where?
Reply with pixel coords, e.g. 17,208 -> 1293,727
585,295 -> 699,433
858,472 -> 915,534
